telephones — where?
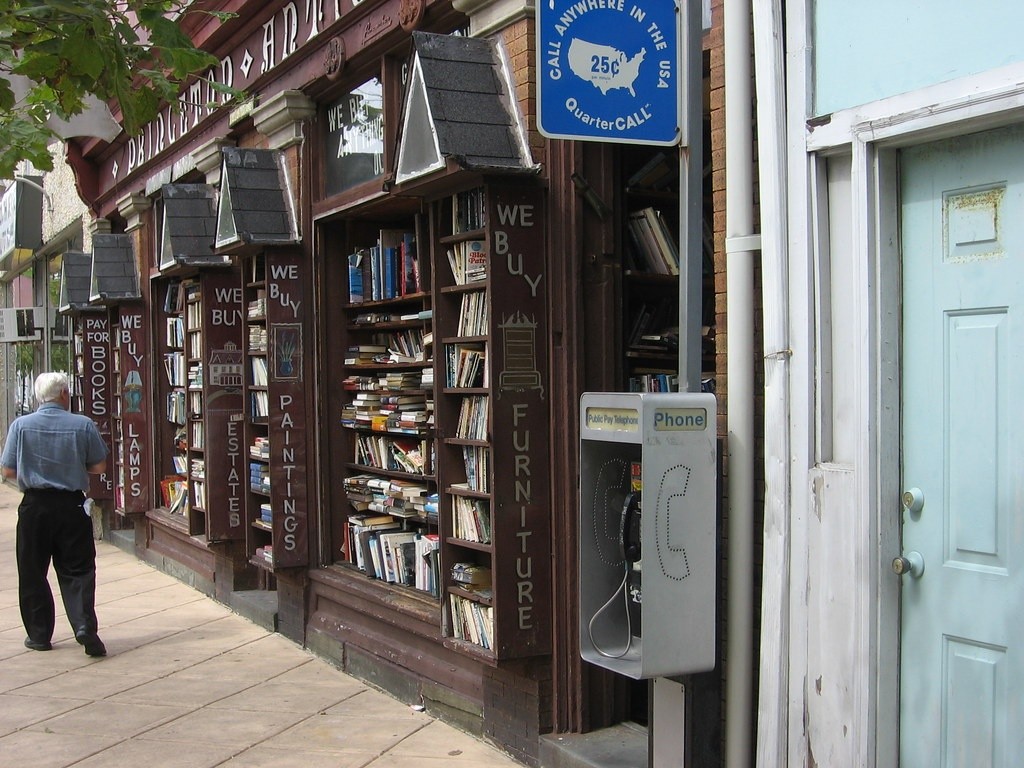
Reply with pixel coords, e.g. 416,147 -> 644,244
618,460 -> 643,574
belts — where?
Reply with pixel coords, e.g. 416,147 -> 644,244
24,488 -> 46,494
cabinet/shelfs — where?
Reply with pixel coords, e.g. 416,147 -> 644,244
312,182 -> 548,665
108,303 -> 149,515
72,310 -> 116,500
151,277 -> 244,546
238,247 -> 312,574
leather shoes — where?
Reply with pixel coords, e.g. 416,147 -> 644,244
24,636 -> 52,651
76,626 -> 107,657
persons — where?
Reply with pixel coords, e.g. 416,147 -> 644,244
2,372 -> 110,657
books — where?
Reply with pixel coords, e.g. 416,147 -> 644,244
163,279 -> 205,516
442,186 -> 493,651
74,335 -> 85,413
338,227 -> 439,596
115,327 -> 124,508
620,151 -> 716,393
247,298 -> 272,564
